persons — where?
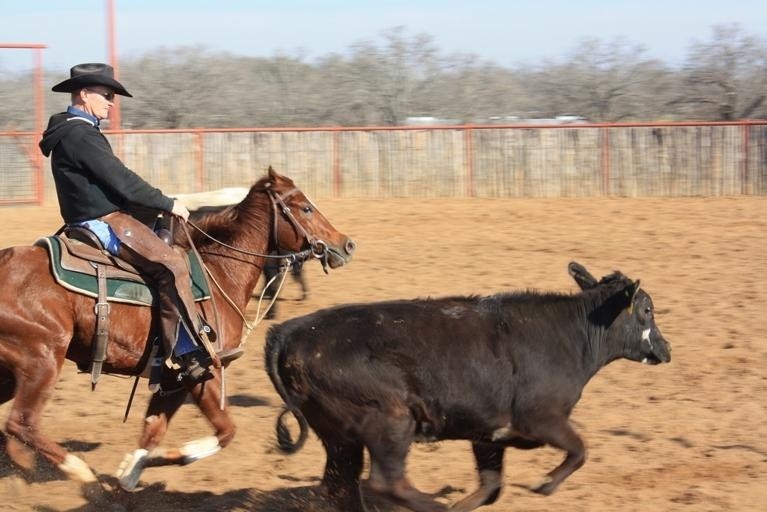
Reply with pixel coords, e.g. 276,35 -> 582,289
39,64 -> 245,395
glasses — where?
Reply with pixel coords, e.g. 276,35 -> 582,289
87,88 -> 114,100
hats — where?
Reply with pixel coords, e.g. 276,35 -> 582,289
52,63 -> 133,98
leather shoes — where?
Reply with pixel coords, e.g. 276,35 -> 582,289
159,368 -> 215,391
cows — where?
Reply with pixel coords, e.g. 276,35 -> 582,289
262,257 -> 675,512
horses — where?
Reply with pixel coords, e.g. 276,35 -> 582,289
0,161 -> 358,497
